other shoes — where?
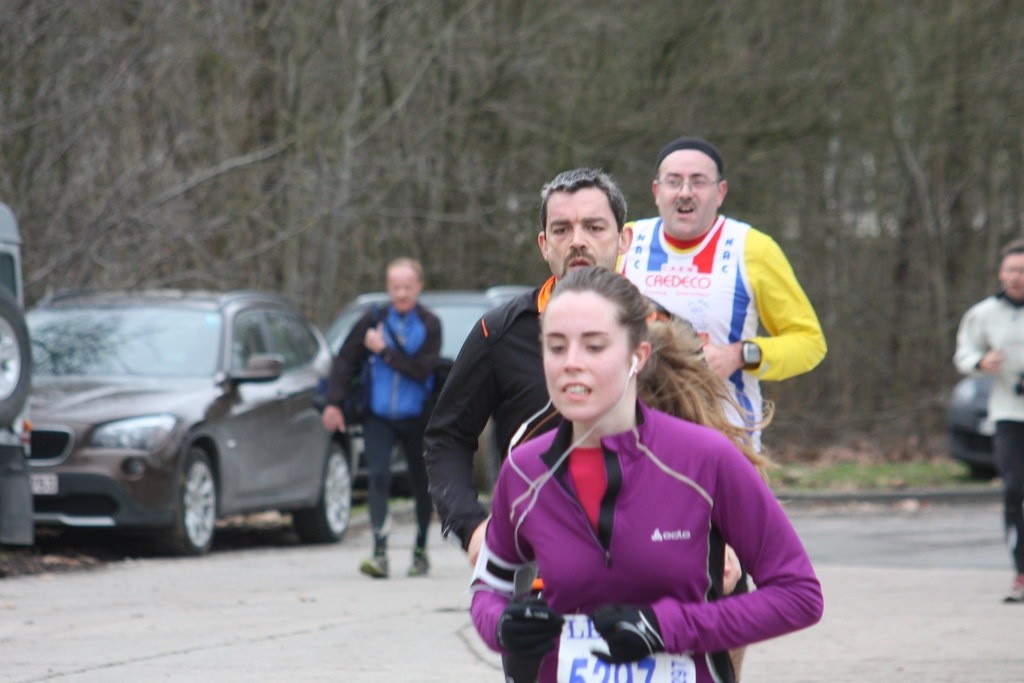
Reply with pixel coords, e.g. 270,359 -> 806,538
1005,573 -> 1024,601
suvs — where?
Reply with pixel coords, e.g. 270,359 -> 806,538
317,286 -> 539,499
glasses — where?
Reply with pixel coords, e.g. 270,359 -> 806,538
658,173 -> 719,190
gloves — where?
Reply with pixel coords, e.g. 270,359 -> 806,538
591,605 -> 666,663
497,596 -> 565,658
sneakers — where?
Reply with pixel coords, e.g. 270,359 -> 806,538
409,560 -> 428,576
361,559 -> 388,578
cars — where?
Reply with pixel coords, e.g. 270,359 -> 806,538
941,367 -> 997,479
19,286 -> 351,557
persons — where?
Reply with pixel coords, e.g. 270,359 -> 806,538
951,239 -> 1024,602
425,167 -> 746,597
612,137 -> 829,683
320,256 -> 444,578
465,264 -> 824,683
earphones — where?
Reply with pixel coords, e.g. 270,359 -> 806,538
628,354 -> 638,378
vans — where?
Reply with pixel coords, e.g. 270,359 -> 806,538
0,197 -> 34,548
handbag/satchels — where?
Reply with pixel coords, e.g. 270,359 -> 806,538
312,309 -> 379,424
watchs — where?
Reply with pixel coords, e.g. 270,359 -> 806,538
742,341 -> 762,370
